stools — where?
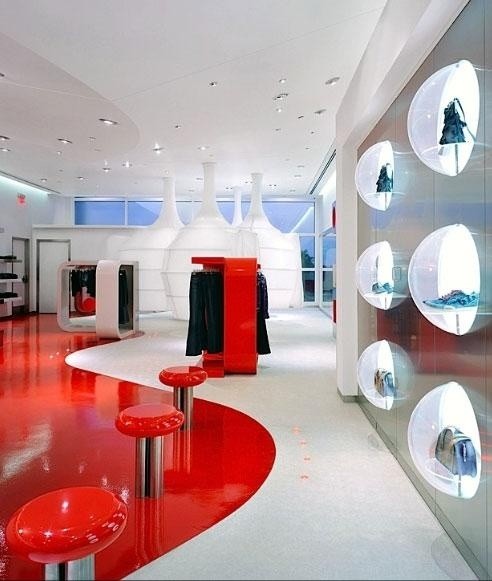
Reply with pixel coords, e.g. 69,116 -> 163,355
6,489 -> 128,581
159,367 -> 206,431
115,402 -> 183,499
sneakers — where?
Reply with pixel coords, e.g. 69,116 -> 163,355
371,281 -> 385,294
421,289 -> 480,311
383,283 -> 393,294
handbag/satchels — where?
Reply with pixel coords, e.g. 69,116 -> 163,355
374,367 -> 394,398
439,97 -> 476,145
432,425 -> 478,497
375,161 -> 393,195
